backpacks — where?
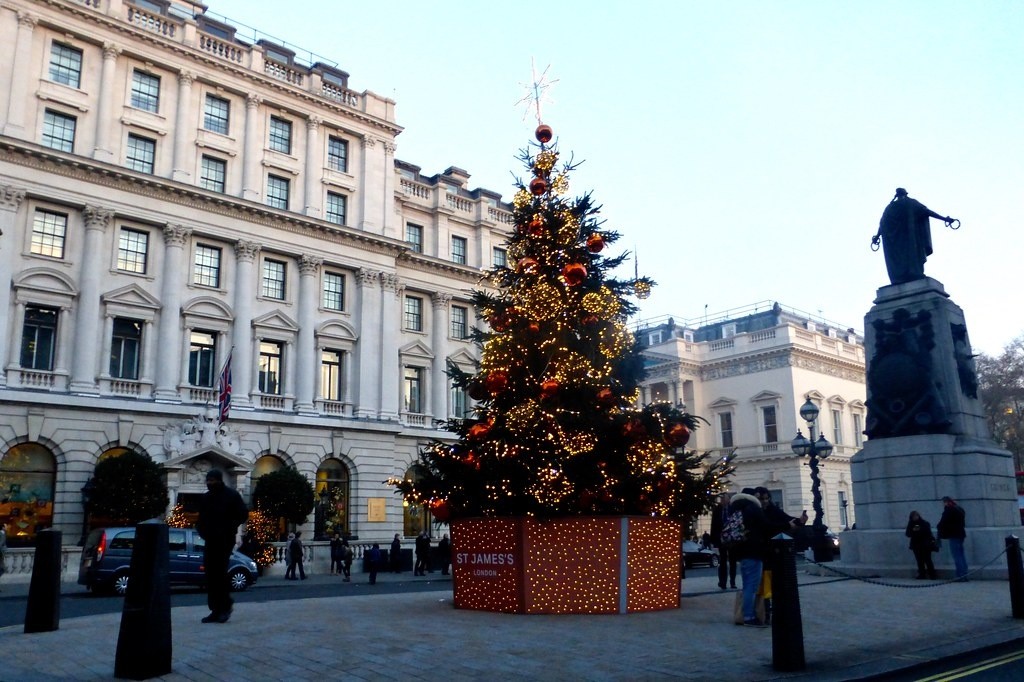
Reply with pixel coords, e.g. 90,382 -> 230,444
720,509 -> 752,547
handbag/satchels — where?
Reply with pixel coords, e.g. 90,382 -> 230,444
930,538 -> 939,553
756,570 -> 772,598
733,593 -> 771,626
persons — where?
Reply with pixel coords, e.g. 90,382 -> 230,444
693,536 -> 698,543
702,531 -> 711,550
389,534 -> 400,574
937,496 -> 969,582
414,530 -> 434,576
438,534 -> 450,575
870,188 -> 960,281
711,487 -> 808,624
330,533 -> 351,582
906,511 -> 935,580
284,531 -> 307,580
366,544 -> 381,584
196,470 -> 249,622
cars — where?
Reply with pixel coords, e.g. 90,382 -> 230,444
796,524 -> 840,557
682,538 -> 719,569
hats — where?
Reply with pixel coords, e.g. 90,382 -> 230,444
287,533 -> 295,540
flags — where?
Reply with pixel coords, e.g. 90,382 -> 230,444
219,358 -> 231,424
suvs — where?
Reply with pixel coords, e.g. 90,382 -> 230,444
76,527 -> 258,598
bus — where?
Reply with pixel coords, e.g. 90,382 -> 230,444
1015,471 -> 1024,521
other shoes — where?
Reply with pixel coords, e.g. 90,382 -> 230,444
929,573 -> 936,580
732,585 -> 737,589
285,576 -> 291,579
302,576 -> 308,580
420,573 -> 425,576
916,574 -> 927,579
415,572 -> 418,576
292,576 -> 298,580
441,572 -> 450,575
718,583 -> 726,590
743,619 -> 768,628
201,606 -> 233,624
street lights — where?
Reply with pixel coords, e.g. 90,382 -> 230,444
789,393 -> 833,561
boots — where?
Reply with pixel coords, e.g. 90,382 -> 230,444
344,576 -> 350,582
